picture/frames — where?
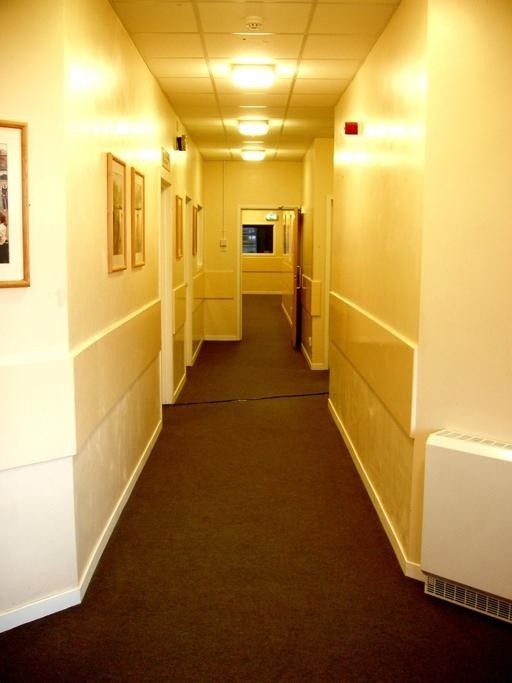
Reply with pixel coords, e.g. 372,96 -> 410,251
192,206 -> 197,255
0,119 -> 30,288
131,167 -> 145,268
176,195 -> 183,258
106,153 -> 128,274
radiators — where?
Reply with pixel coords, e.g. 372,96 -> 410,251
420,427 -> 512,625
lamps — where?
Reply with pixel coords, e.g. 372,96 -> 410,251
222,60 -> 279,92
239,147 -> 266,162
236,118 -> 271,136
